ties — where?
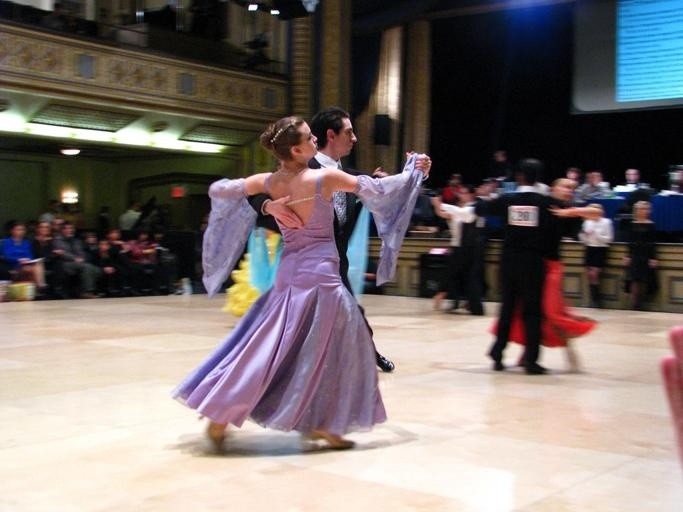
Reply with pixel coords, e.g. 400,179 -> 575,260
334,164 -> 347,227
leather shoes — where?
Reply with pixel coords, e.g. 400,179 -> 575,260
523,363 -> 547,374
377,354 -> 394,372
489,351 -> 503,370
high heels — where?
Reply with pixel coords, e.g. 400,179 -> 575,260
311,431 -> 353,449
198,414 -> 228,448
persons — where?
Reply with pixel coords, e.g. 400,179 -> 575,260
0,198 -> 208,300
660,325 -> 682,464
248,107 -> 431,372
430,149 -> 603,374
171,115 -> 431,448
567,148 -> 683,309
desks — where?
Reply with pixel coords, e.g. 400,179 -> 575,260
576,185 -> 682,238
